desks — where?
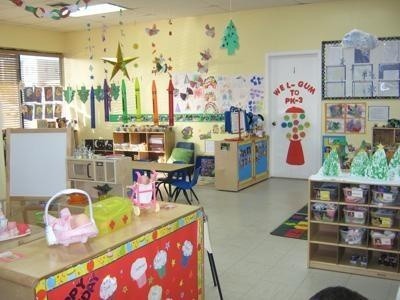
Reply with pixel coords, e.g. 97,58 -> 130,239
130,161 -> 196,202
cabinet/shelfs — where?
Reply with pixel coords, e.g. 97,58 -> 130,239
111,130 -> 175,165
305,172 -> 400,282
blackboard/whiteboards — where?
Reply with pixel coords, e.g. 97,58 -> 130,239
5,128 -> 72,202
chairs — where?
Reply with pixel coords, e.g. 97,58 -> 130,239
168,166 -> 203,205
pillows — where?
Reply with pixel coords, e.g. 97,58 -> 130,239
168,147 -> 196,164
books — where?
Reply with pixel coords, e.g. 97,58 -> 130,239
149,134 -> 163,152
